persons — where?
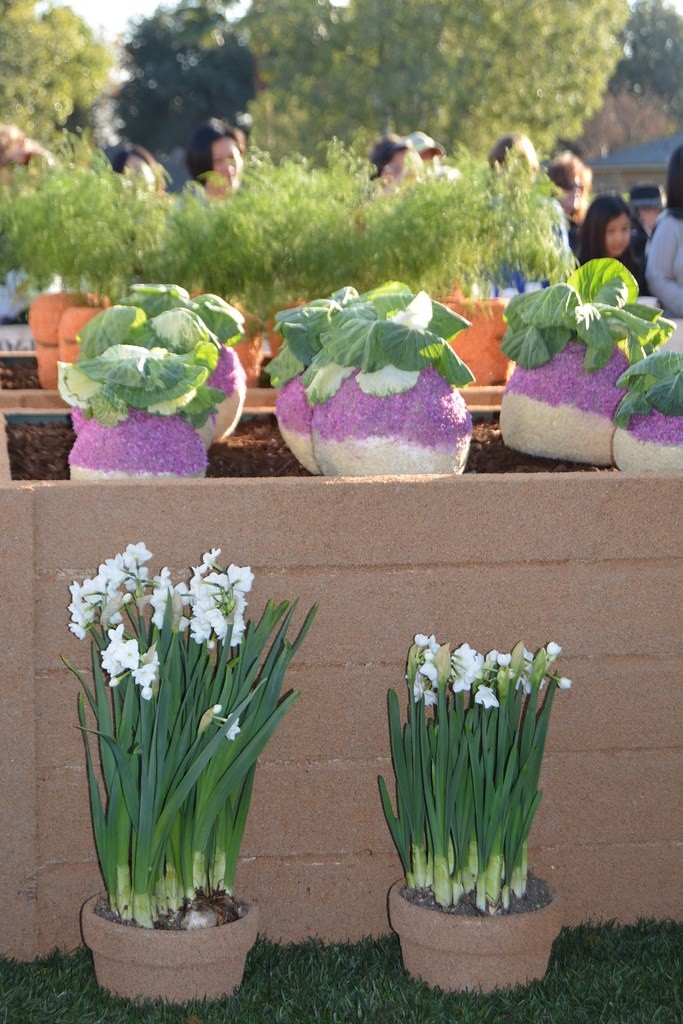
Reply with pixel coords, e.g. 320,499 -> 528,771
0,122 -> 61,325
614,183 -> 663,267
104,141 -> 167,195
487,134 -> 592,258
184,118 -> 250,200
368,130 -> 463,193
576,194 -> 663,310
645,144 -> 683,318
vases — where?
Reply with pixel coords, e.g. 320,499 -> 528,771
388,876 -> 566,995
81,890 -> 260,1006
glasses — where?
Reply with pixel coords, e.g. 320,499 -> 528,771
557,179 -> 585,193
384,163 -> 413,177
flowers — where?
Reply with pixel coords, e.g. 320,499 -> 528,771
378,634 -> 571,914
61,543 -> 319,929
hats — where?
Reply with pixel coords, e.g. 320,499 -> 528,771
0,125 -> 53,169
408,131 -> 444,157
628,181 -> 667,207
